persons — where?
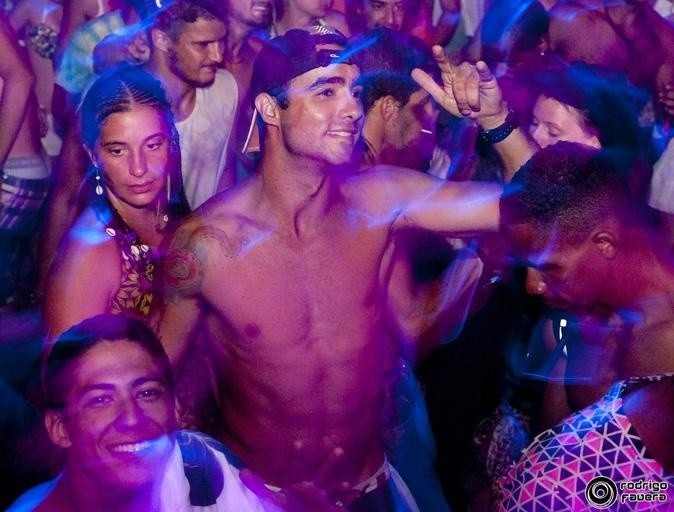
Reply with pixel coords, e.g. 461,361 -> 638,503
1,0 -> 674,507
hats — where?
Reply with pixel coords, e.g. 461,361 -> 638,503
250,25 -> 359,107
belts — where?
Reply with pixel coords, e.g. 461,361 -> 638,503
4,173 -> 49,193
